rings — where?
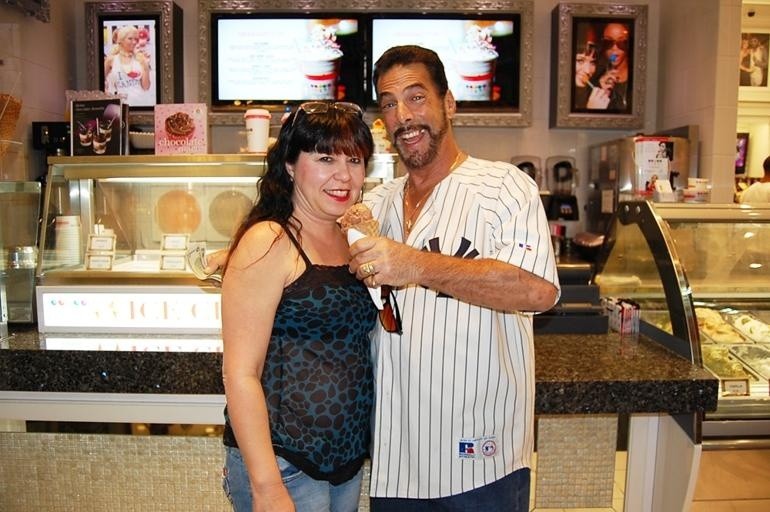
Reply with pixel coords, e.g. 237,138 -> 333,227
361,264 -> 375,275
370,276 -> 377,289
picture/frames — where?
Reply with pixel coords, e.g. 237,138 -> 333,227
545,2 -> 651,132
736,29 -> 770,102
84,0 -> 183,127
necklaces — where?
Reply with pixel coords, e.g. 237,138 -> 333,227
402,148 -> 463,232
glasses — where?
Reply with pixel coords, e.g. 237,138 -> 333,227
601,40 -> 628,52
375,285 -> 404,338
291,99 -> 364,127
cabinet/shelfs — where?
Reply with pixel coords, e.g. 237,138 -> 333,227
588,182 -> 770,450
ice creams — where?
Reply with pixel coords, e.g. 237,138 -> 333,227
302,24 -> 341,60
340,203 -> 379,249
455,25 -> 498,60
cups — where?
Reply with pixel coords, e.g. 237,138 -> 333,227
78,121 -> 114,154
243,108 -> 271,153
297,45 -> 344,99
461,48 -> 499,103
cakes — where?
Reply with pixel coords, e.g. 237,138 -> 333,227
164,112 -> 195,137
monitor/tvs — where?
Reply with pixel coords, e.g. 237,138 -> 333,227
210,10 -> 366,112
366,9 -> 524,112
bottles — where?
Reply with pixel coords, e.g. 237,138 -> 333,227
54,214 -> 83,266
681,178 -> 708,201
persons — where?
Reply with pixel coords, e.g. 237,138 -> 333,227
198,46 -> 563,512
740,37 -> 753,85
574,34 -> 609,112
749,35 -> 769,86
647,175 -> 658,190
596,21 -> 628,111
216,101 -> 379,512
737,156 -> 770,204
103,25 -> 151,107
657,142 -> 672,160
